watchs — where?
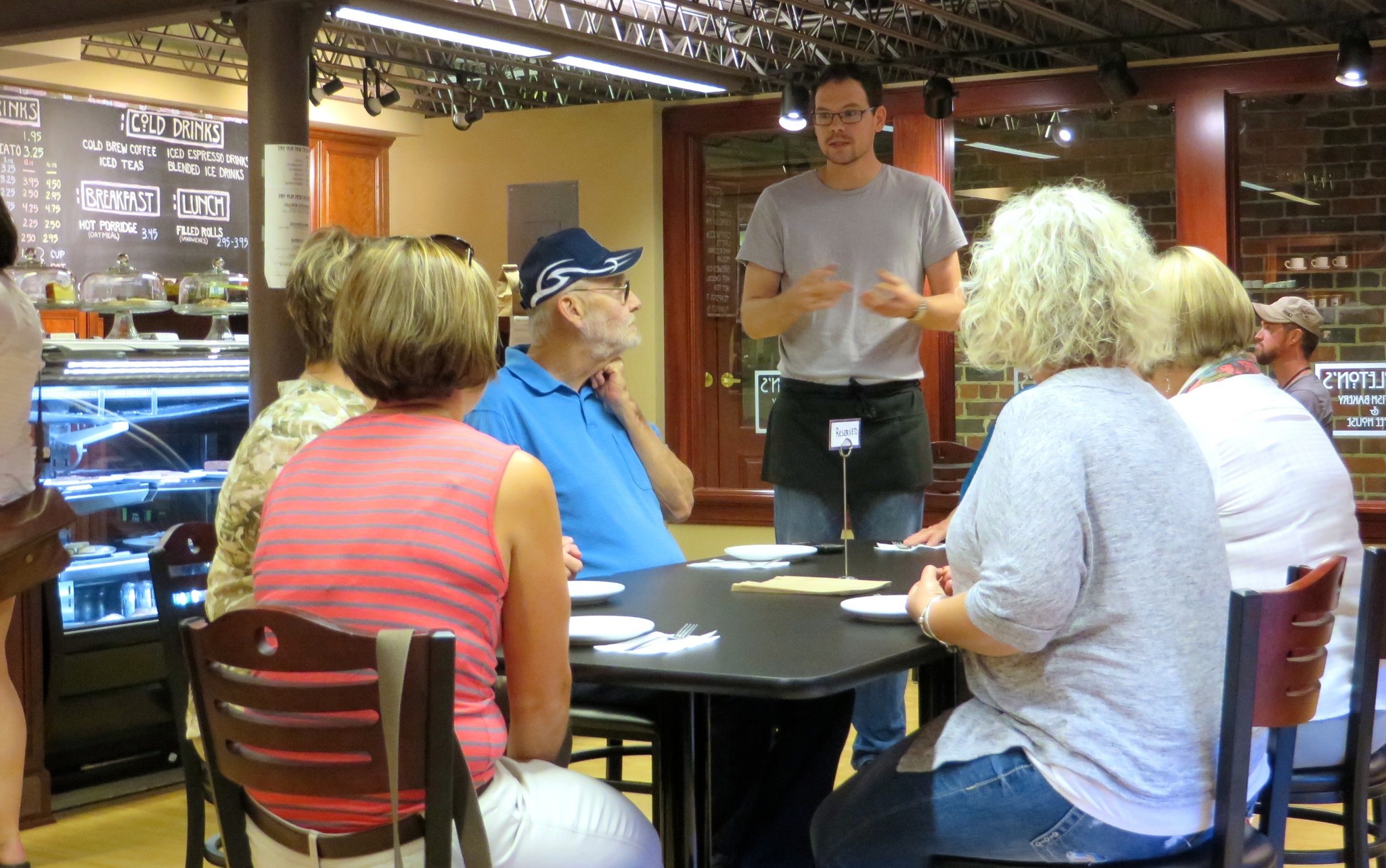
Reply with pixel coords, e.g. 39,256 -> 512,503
908,298 -> 928,322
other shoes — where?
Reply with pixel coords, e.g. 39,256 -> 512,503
0,860 -> 31,868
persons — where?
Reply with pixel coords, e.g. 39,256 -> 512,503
464,226 -> 694,578
810,181 -> 1270,868
185,224 -> 381,772
0,194 -> 44,868
735,63 -> 968,774
1252,296 -> 1334,440
904,243 -> 1386,765
250,234 -> 664,868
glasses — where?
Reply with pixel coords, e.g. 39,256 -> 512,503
809,107 -> 877,125
567,280 -> 632,307
389,232 -> 474,271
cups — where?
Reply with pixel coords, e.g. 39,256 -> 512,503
1332,256 -> 1346,266
1285,258 -> 1304,268
1311,257 -> 1329,266
1243,279 -> 1297,288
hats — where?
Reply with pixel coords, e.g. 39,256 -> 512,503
520,227 -> 645,311
1252,296 -> 1324,339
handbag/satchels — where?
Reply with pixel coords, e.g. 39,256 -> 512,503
0,490 -> 76,602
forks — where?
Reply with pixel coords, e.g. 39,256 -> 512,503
625,623 -> 698,650
707,558 -> 783,566
873,593 -> 882,596
891,541 -> 944,550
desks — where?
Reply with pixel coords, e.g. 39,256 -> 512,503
494,540 -> 972,868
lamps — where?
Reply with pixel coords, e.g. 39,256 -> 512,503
363,65 -> 400,116
307,50 -> 344,107
923,71 -> 953,120
1098,61 -> 1138,106
1334,22 -> 1373,89
778,77 -> 810,131
452,74 -> 485,131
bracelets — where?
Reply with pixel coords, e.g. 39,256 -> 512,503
917,604 -> 958,654
1282,367 -> 1311,389
925,594 -> 949,646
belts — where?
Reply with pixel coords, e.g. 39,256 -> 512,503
239,779 -> 493,860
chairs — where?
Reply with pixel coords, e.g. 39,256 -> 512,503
920,542 -> 1386,868
148,520 -> 455,868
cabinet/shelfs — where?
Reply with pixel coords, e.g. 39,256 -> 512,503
38,370 -> 250,797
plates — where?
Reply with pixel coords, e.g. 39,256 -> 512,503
724,544 -> 818,562
1333,265 -> 1348,269
569,615 -> 655,644
567,579 -> 626,606
840,595 -> 915,623
1287,267 -> 1307,270
68,544 -> 117,561
122,537 -> 158,552
1313,266 -> 1331,268
